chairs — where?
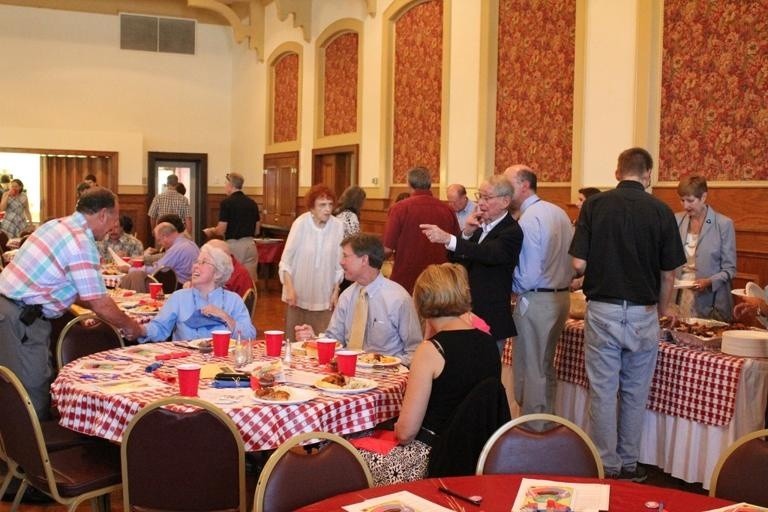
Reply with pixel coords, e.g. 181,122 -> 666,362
709,427 -> 767,509
119,396 -> 245,511
1,224 -> 768,492
1,365 -> 123,511
255,433 -> 373,512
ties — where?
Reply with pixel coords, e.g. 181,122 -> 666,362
346,288 -> 368,352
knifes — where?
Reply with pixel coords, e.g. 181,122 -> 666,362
173,343 -> 194,350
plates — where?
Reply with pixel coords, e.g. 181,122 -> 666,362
718,331 -> 768,359
187,337 -> 236,352
107,291 -> 162,316
98,271 -> 124,277
293,339 -> 342,351
250,385 -> 317,404
672,279 -> 697,288
315,375 -> 378,393
730,281 -> 766,326
356,355 -> 404,367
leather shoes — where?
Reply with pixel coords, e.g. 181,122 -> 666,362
1,483 -> 53,501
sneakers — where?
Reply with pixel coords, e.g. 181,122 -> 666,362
619,464 -> 649,483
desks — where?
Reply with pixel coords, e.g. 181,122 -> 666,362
302,474 -> 763,511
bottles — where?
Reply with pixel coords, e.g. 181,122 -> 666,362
284,338 -> 293,362
233,330 -> 254,364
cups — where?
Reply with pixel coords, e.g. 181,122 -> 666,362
176,363 -> 201,396
264,329 -> 285,357
336,351 -> 360,375
148,283 -> 165,300
121,256 -> 144,268
316,338 -> 336,365
211,329 -> 232,357
12,189 -> 16,195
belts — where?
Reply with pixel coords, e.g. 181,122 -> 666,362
531,287 -> 570,292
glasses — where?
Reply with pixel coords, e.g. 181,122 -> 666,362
225,173 -> 230,181
474,192 -> 504,201
194,259 -> 216,266
647,168 -> 651,189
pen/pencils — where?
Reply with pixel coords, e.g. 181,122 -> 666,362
439,486 -> 481,505
